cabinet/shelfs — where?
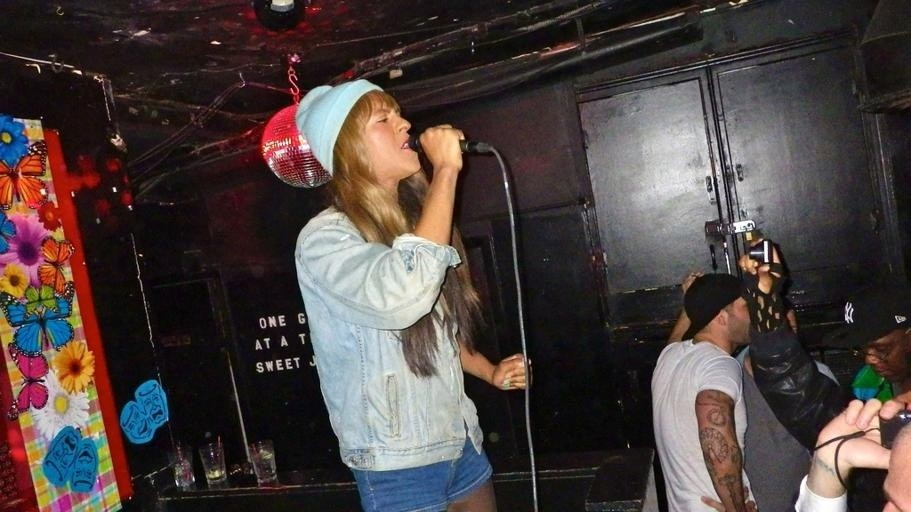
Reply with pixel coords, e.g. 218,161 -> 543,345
572,1 -> 903,351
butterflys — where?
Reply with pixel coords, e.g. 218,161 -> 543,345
38,233 -> 75,293
6,342 -> 49,422
0,139 -> 48,210
0,279 -> 75,359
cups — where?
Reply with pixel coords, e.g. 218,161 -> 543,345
167,439 -> 277,487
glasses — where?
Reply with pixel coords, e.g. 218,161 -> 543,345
849,330 -> 911,360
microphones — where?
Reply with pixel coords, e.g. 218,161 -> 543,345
406,131 -> 495,157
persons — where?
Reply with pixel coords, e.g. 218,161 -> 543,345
292,77 -> 538,511
735,298 -> 845,511
738,230 -> 911,512
650,272 -> 758,510
791,388 -> 911,512
835,271 -> 911,403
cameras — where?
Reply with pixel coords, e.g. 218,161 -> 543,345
877,407 -> 910,449
749,237 -> 773,265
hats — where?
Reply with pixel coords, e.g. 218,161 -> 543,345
677,270 -> 741,343
821,280 -> 911,351
294,78 -> 385,182
